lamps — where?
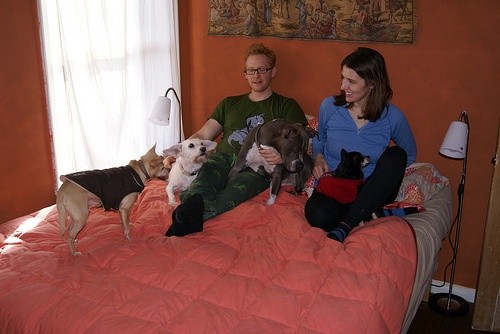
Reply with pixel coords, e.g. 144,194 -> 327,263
427,109 -> 472,317
148,87 -> 181,144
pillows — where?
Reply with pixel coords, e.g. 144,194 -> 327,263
280,115 -> 451,206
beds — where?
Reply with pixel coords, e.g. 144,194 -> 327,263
0,150 -> 452,334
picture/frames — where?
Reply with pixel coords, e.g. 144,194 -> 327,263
206,0 -> 418,45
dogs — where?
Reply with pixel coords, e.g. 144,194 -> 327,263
226,118 -> 320,207
305,147 -> 371,231
162,139 -> 218,205
56,141 -> 164,256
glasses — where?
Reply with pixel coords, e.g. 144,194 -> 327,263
244,67 -> 273,75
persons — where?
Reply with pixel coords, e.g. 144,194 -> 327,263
162,45 -> 308,236
304,47 -> 417,242
